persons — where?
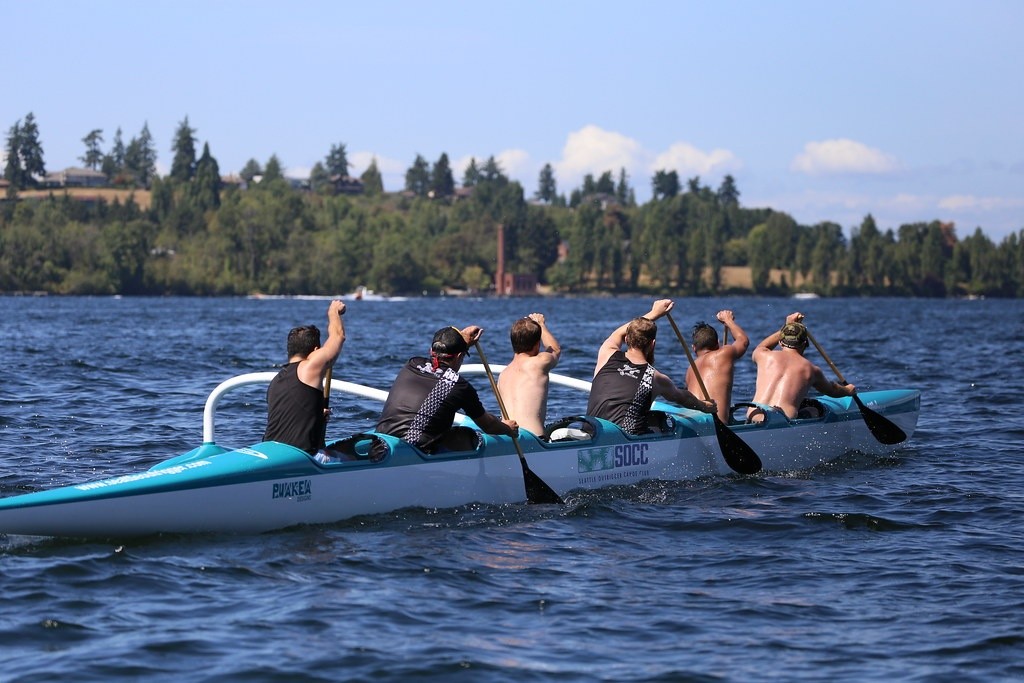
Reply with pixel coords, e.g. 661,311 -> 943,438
496,313 -> 561,443
582,299 -> 718,436
746,312 -> 857,425
261,300 -> 357,463
685,310 -> 750,426
368,326 -> 519,463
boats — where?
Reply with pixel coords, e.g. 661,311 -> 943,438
0,365 -> 923,540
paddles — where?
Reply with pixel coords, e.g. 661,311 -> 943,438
800,322 -> 907,444
324,308 -> 340,429
718,316 -> 736,345
664,308 -> 762,475
473,339 -> 564,505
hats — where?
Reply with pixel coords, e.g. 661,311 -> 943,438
432,327 -> 470,358
781,323 -> 809,349
